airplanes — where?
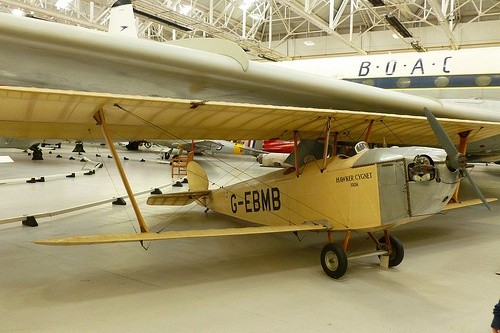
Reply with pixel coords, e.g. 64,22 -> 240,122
142,137 -> 273,166
0,0 -> 499,281
